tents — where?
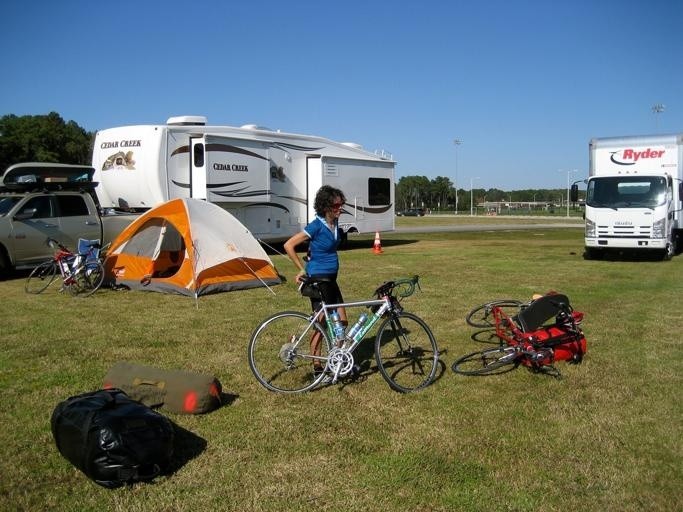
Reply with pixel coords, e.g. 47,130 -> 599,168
101,198 -> 287,309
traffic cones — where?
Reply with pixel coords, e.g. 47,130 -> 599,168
371,228 -> 386,253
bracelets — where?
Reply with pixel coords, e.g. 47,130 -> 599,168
299,268 -> 305,272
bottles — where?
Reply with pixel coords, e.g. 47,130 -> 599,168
330,310 -> 345,340
346,313 -> 369,341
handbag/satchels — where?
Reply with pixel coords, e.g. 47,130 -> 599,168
299,278 -> 329,298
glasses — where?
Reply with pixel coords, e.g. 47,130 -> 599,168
326,202 -> 344,209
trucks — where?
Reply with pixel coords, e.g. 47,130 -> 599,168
571,136 -> 682,263
83,114 -> 397,251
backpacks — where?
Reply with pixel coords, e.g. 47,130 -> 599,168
97,363 -> 224,415
51,386 -> 177,489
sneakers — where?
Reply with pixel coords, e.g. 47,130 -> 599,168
313,372 -> 338,385
345,360 -> 365,376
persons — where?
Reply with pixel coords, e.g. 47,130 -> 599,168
284,186 -> 349,383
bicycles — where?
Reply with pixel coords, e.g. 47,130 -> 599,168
24,236 -> 105,299
450,289 -> 587,377
242,274 -> 439,396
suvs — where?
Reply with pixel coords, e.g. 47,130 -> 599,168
397,208 -> 425,217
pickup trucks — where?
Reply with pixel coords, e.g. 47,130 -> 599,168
0,193 -> 169,275
